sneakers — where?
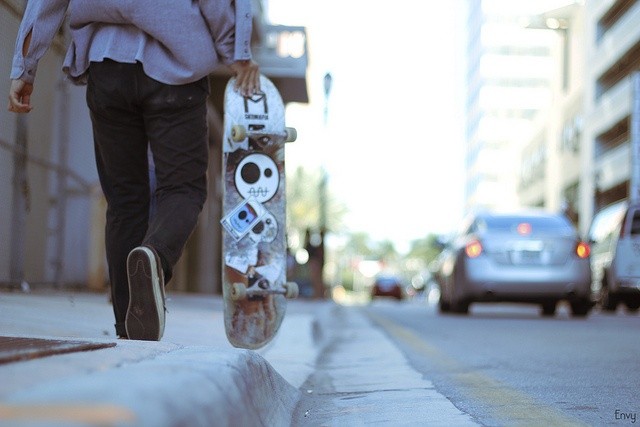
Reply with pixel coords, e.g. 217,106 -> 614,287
125,244 -> 165,341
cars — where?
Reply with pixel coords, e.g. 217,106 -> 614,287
373,278 -> 402,300
440,212 -> 592,317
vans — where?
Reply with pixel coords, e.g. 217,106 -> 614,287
589,198 -> 640,311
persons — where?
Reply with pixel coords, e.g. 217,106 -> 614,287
6,1 -> 265,341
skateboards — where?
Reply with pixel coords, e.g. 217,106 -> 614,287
221,72 -> 299,350
